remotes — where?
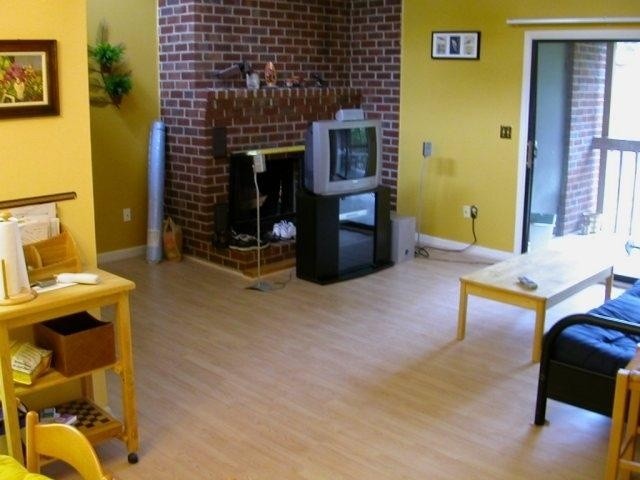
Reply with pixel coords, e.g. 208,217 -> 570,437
518,274 -> 538,290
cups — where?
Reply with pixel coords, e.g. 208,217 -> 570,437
246,71 -> 261,90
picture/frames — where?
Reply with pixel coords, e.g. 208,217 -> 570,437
431,31 -> 481,60
0,38 -> 60,119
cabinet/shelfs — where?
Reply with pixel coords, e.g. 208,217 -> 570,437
604,352 -> 640,480
1,267 -> 140,473
295,185 -> 395,285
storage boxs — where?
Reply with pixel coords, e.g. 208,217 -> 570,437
33,309 -> 116,377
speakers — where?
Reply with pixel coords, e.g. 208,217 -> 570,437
253,154 -> 265,173
422,141 -> 432,158
389,210 -> 416,263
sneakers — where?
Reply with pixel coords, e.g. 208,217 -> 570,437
271,220 -> 297,239
230,234 -> 271,251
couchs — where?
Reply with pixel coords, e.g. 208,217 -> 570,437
535,276 -> 640,426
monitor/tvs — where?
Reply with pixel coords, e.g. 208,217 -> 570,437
303,118 -> 383,197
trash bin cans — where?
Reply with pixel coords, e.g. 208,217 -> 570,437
582,211 -> 603,234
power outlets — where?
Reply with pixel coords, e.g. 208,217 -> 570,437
123,207 -> 133,222
462,205 -> 478,219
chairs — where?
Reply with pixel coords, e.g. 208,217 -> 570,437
25,411 -> 116,480
